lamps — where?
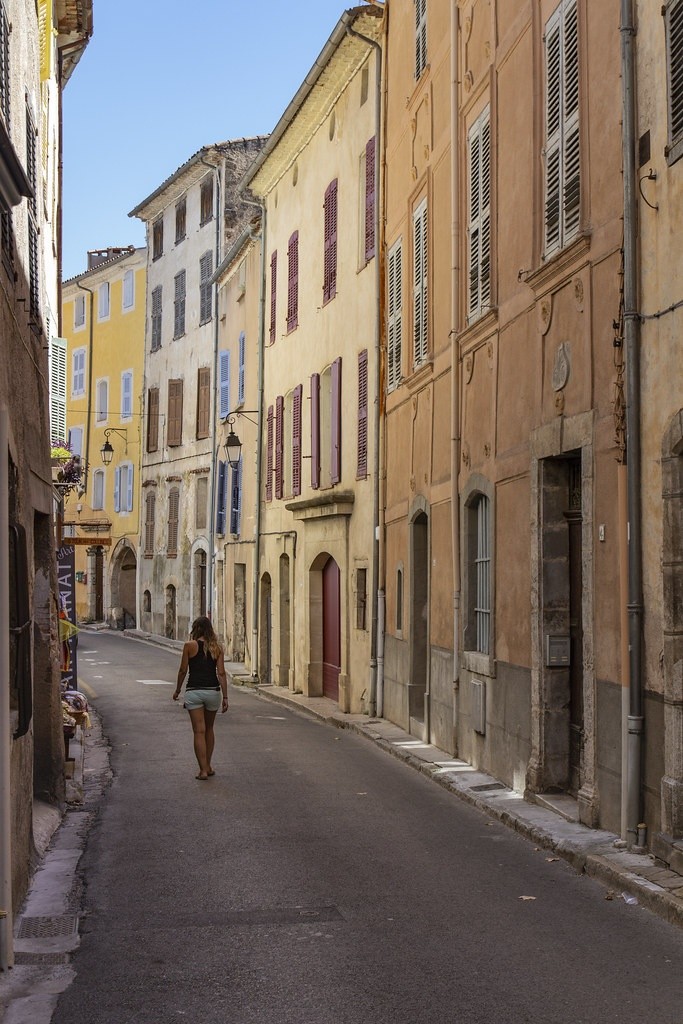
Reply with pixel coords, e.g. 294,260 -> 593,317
223,410 -> 258,469
99,427 -> 127,466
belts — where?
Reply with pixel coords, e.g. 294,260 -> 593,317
185,687 -> 220,691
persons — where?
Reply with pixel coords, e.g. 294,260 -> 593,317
173,616 -> 228,780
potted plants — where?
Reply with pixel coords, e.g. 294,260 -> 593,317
50,447 -> 71,480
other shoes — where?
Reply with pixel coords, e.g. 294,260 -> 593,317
196,774 -> 207,780
207,770 -> 215,776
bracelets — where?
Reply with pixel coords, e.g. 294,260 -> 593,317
176,690 -> 181,693
223,697 -> 228,699
